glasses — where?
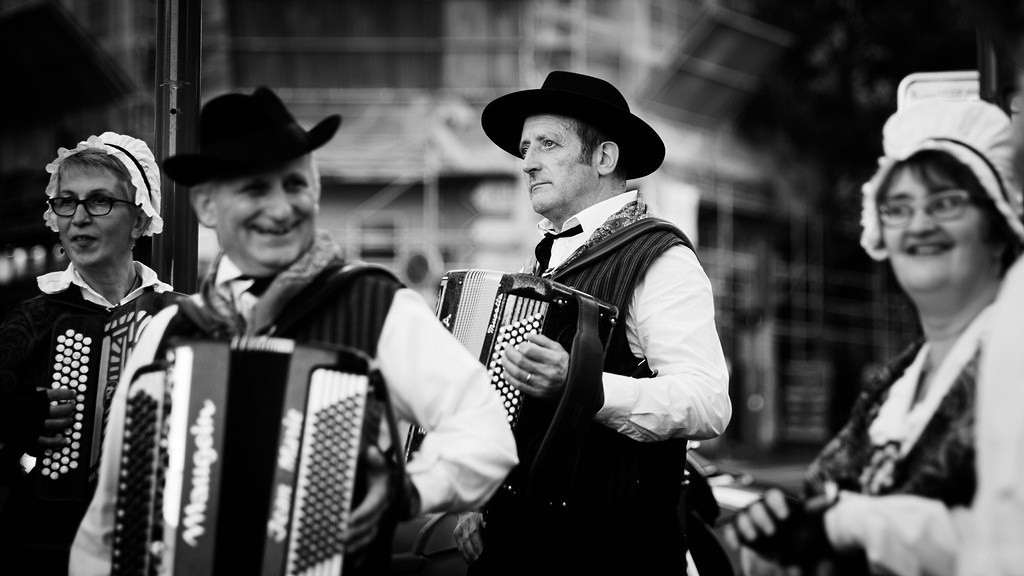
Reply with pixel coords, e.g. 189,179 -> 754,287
49,195 -> 142,216
879,189 -> 980,227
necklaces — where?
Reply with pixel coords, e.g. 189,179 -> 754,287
125,271 -> 137,297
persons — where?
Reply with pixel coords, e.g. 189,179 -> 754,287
68,85 -> 520,576
730,95 -> 1024,576
452,71 -> 732,576
0,132 -> 175,576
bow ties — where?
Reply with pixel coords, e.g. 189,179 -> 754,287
535,224 -> 584,277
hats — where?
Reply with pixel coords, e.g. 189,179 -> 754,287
481,70 -> 665,180
162,85 -> 343,187
860,99 -> 1024,262
43,131 -> 164,237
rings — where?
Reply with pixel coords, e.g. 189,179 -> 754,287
527,373 -> 531,384
